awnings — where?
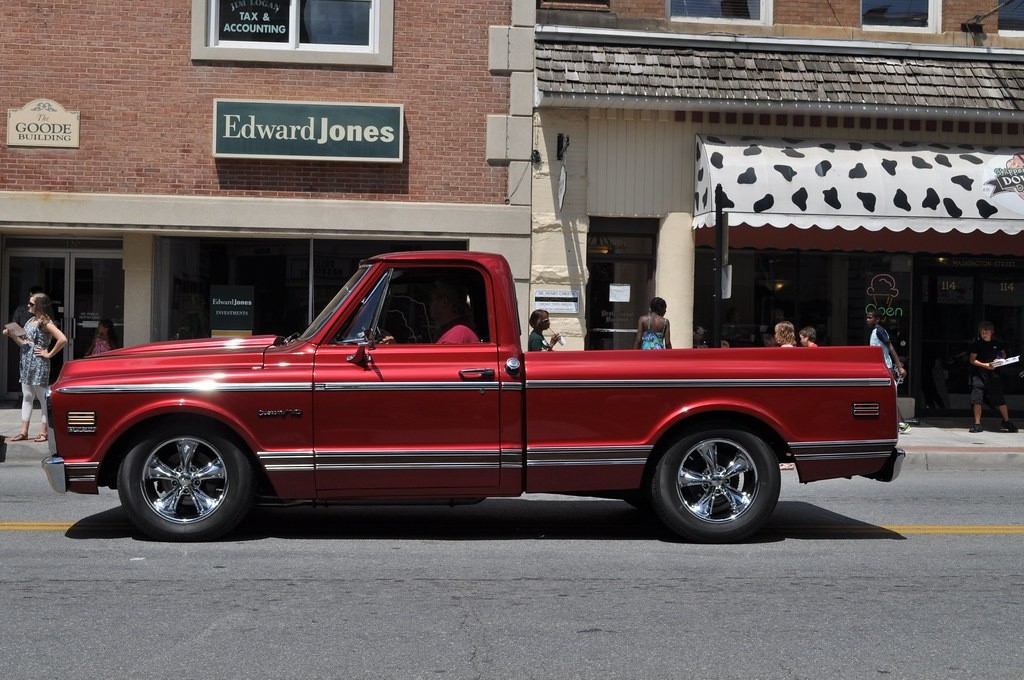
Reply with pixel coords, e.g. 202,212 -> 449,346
693,133 -> 1024,236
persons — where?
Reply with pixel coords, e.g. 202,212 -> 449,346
634,297 -> 672,349
529,309 -> 561,352
12,286 -> 49,409
379,281 -> 482,344
969,322 -> 1018,433
85,318 -> 117,358
866,308 -> 912,434
924,352 -> 952,416
693,306 -> 818,348
3,292 -> 68,442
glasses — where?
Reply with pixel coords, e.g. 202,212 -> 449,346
541,319 -> 549,323
28,302 -> 35,307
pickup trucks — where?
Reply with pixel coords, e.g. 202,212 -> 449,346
40,250 -> 906,544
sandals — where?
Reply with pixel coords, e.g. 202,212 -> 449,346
10,432 -> 28,441
34,433 -> 48,441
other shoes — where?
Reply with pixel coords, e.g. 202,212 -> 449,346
899,423 -> 911,434
779,463 -> 794,470
16,396 -> 22,409
969,423 -> 983,432
1000,419 -> 1018,432
